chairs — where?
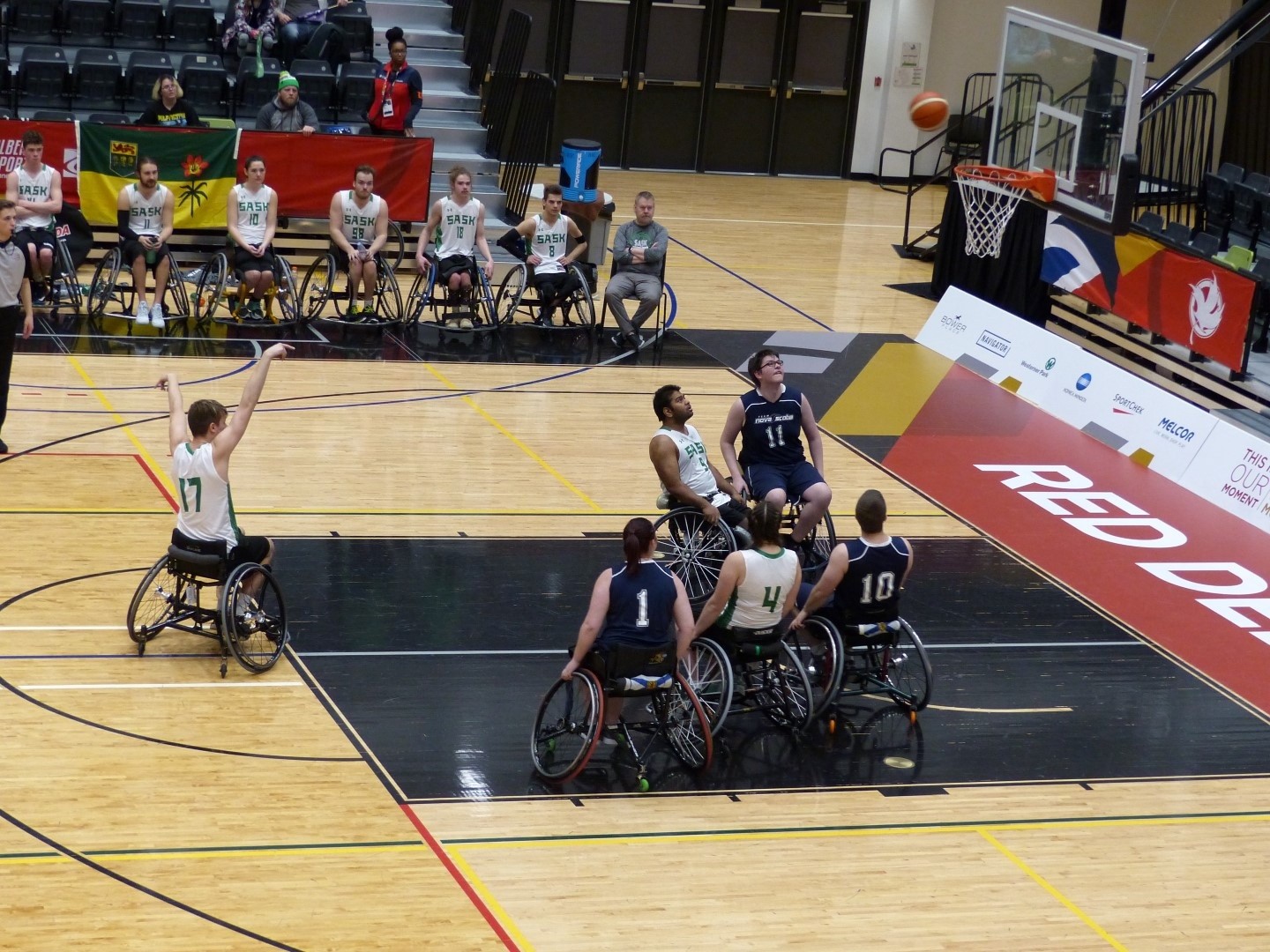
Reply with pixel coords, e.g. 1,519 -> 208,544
932,114 -> 985,183
1131,162 -> 1270,354
0,0 -> 385,135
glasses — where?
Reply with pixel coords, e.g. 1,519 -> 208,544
758,359 -> 783,369
162,85 -> 178,91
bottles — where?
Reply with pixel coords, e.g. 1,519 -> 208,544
146,237 -> 158,263
191,292 -> 205,306
280,273 -> 289,300
357,242 -> 368,262
291,267 -> 298,288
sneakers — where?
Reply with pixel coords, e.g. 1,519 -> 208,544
362,305 -> 384,324
151,303 -> 165,328
234,298 -> 250,319
136,303 -> 150,325
247,300 -> 264,321
346,305 -> 367,323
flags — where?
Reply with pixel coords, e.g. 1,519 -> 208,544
0,117 -> 436,233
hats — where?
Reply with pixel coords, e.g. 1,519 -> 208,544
278,70 -> 299,91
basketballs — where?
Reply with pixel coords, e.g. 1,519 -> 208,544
908,91 -> 951,132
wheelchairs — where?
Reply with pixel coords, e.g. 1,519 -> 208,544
15,216 -> 83,314
87,227 -> 190,320
299,237 -> 403,326
126,528 -> 288,679
190,232 -> 299,328
654,474 -> 837,604
530,612 -> 934,795
495,240 -> 596,332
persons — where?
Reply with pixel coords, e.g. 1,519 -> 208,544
497,185 -> 587,329
327,164 -> 389,318
360,25 -> 424,139
5,130 -> 63,302
225,0 -> 350,113
0,199 -> 33,455
113,152 -> 174,328
561,347 -> 914,746
227,154 -> 279,319
154,342 -> 295,630
134,70 -> 203,127
604,191 -> 668,346
414,166 -> 495,328
256,68 -> 319,231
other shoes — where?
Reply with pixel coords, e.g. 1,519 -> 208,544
536,308 -> 554,327
577,722 -> 601,745
209,611 -> 258,631
602,729 -> 619,744
611,332 -> 625,348
447,307 -> 460,327
626,334 -> 645,352
460,305 -> 474,328
37,276 -> 55,302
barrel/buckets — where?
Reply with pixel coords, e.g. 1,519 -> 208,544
559,139 -> 601,203
